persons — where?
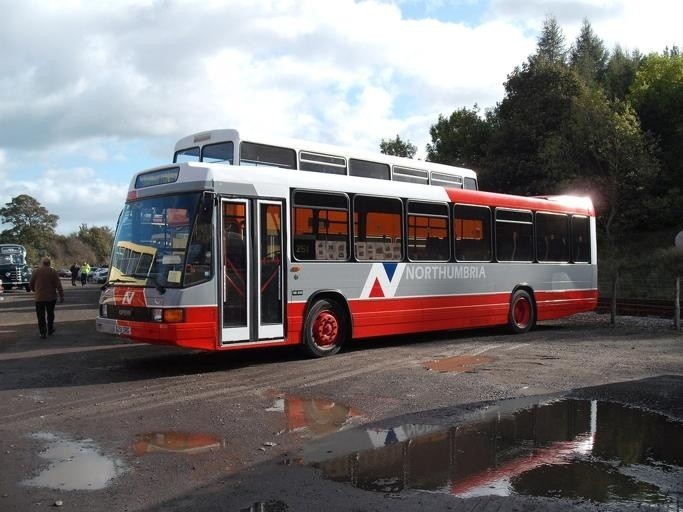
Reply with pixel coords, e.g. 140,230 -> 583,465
70,262 -> 80,286
30,258 -> 65,339
80,261 -> 90,287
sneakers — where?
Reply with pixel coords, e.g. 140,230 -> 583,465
48,327 -> 56,335
39,334 -> 47,338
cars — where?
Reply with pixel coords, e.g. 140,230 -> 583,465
56,267 -> 108,284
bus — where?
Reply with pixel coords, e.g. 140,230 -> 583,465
0,244 -> 33,292
133,394 -> 598,511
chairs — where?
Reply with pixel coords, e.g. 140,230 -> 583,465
505,232 -> 568,259
